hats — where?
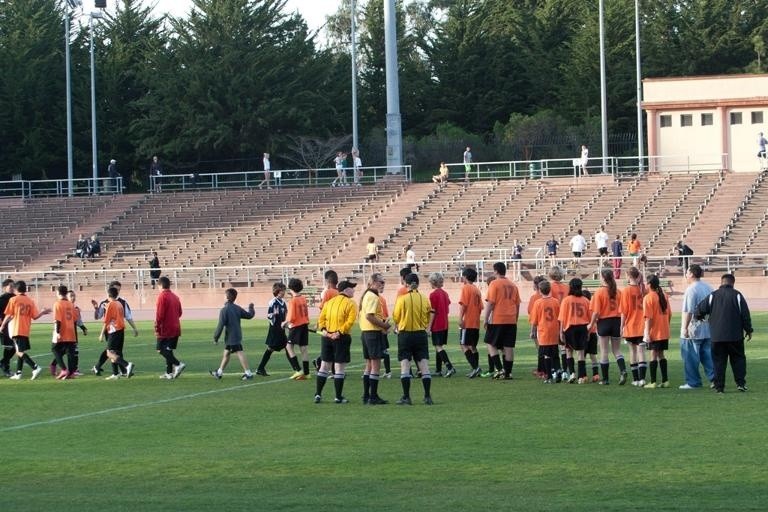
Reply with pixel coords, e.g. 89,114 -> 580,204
336,280 -> 356,291
403,274 -> 418,285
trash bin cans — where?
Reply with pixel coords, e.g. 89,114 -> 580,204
529,164 -> 538,180
103,179 -> 112,195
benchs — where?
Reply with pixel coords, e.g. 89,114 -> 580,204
0,168 -> 768,307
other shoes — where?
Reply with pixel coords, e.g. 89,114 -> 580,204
414,370 -> 422,378
409,373 -> 413,378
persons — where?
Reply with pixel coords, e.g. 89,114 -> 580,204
458,268 -> 484,377
694,274 -> 753,393
527,265 -> 647,386
208,288 -> 256,380
51,285 -> 81,380
48,289 -> 88,376
429,273 -> 456,378
332,152 -> 345,188
363,236 -> 381,270
72,234 -> 103,261
643,274 -> 672,388
546,227 -> 694,279
431,161 -> 449,189
154,276 -> 186,380
679,264 -> 715,388
257,283 -> 296,376
754,130 -> 768,173
580,144 -> 590,175
313,270 -> 358,403
337,153 -> 351,188
146,251 -> 163,288
403,245 -> 418,271
257,152 -> 273,190
358,273 -> 392,404
352,146 -> 363,187
0,278 -> 18,377
150,156 -> 166,194
0,280 -> 54,381
107,158 -> 121,194
91,281 -> 135,377
463,144 -> 474,182
392,268 -> 433,405
98,288 -> 135,380
511,238 -> 527,283
480,262 -> 520,380
281,278 -> 310,379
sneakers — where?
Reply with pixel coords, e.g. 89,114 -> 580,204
172,361 -> 186,379
160,372 -> 173,379
532,368 -> 748,393
314,393 -> 434,404
30,366 -> 41,379
431,372 -> 442,377
444,369 -> 456,377
0,361 -> 10,374
289,369 -> 304,379
379,371 -> 392,379
465,368 -> 513,380
93,365 -> 101,376
49,363 -> 83,381
104,363 -> 134,380
241,373 -> 253,381
296,373 -> 311,380
209,368 -> 222,380
256,368 -> 270,376
10,371 -> 21,380
